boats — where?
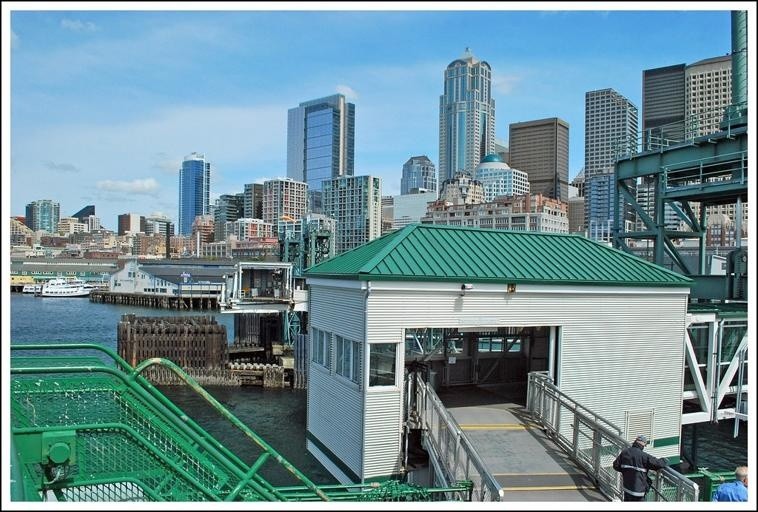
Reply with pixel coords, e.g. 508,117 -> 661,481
23,276 -> 98,297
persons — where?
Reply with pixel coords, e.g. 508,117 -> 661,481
613,435 -> 671,501
712,466 -> 748,502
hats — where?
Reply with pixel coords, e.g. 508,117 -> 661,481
636,435 -> 647,446
